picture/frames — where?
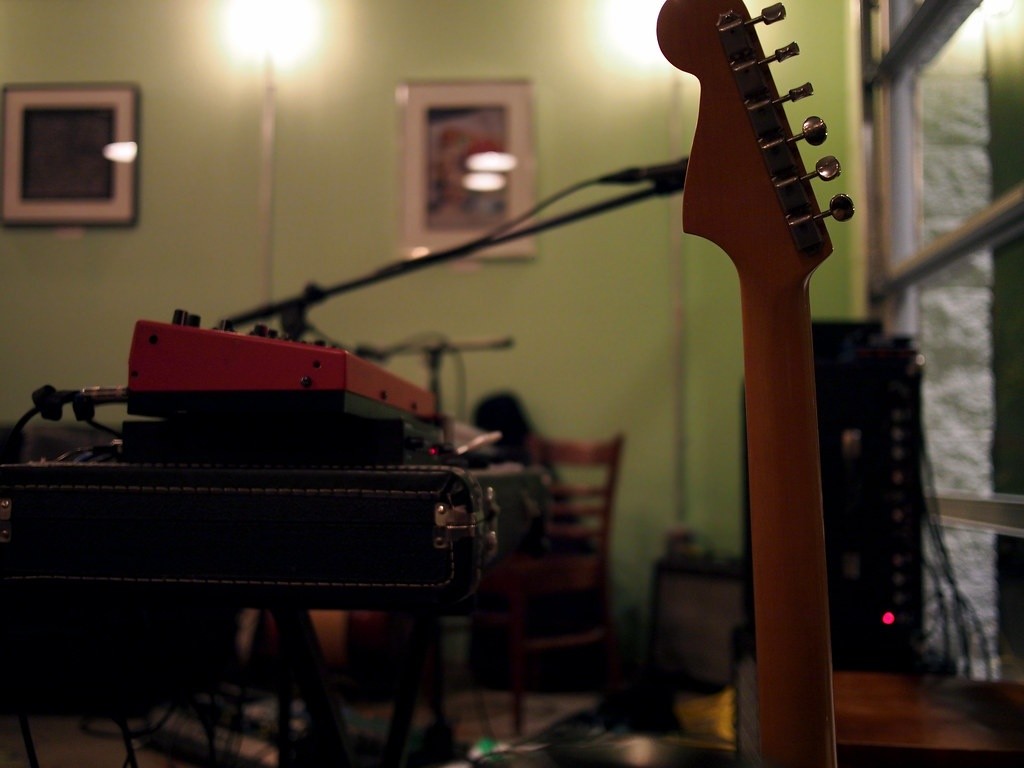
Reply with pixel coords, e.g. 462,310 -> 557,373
399,76 -> 536,261
2,84 -> 142,229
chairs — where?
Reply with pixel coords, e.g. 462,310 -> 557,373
453,432 -> 625,730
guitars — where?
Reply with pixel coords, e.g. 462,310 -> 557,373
654,0 -> 854,767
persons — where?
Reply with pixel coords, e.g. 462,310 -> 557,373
463,393 -> 600,677
597,561 -> 735,732
